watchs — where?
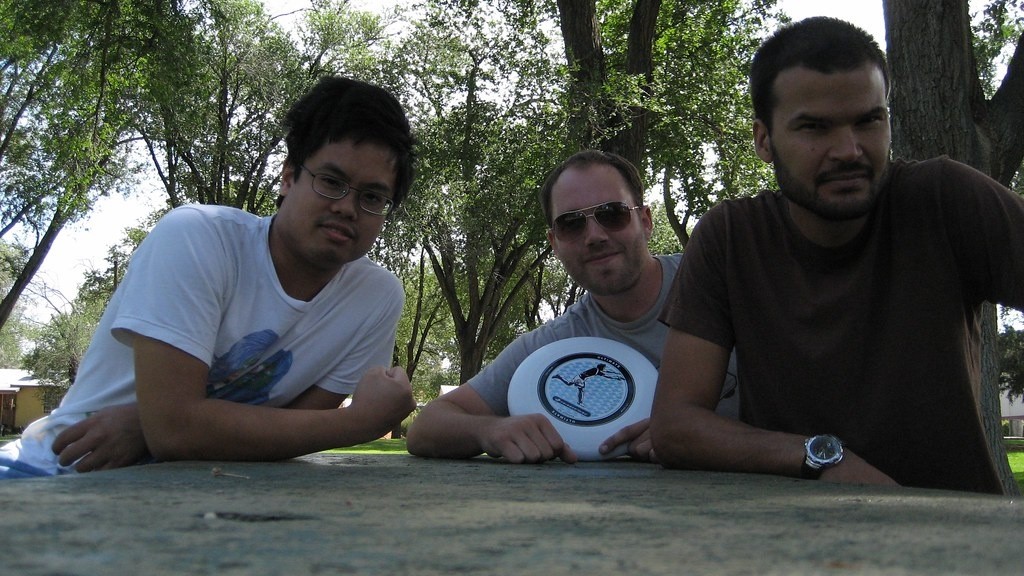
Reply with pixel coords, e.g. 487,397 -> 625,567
802,434 -> 844,481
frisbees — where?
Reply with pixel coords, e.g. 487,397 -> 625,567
507,336 -> 660,463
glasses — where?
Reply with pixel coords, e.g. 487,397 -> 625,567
550,201 -> 641,242
299,163 -> 395,216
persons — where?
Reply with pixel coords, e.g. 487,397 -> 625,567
0,73 -> 416,480
649,15 -> 1024,498
405,149 -> 740,465
